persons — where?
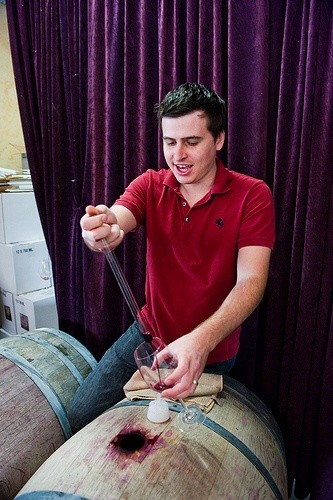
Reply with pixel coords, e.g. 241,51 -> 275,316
68,82 -> 275,430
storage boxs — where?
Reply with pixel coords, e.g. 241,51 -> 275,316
0,193 -> 58,338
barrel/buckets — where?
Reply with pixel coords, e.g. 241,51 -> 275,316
0,328 -> 97,500
14,375 -> 289,500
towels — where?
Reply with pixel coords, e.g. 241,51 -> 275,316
123,365 -> 223,414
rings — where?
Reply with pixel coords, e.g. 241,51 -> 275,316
192,380 -> 199,385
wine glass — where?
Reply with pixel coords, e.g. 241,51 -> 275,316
39,262 -> 53,295
134,338 -> 207,431
